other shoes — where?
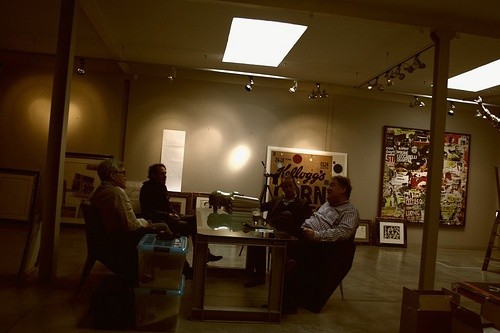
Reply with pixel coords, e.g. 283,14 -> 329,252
247,271 -> 266,287
206,253 -> 223,263
282,303 -> 298,315
183,267 -> 193,276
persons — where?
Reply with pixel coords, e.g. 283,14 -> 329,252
94,160 -> 223,295
260,176 -> 362,315
235,175 -> 313,286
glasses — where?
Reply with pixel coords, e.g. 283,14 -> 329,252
114,170 -> 125,174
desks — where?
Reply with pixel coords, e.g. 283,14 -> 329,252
452,280 -> 500,333
190,207 -> 298,324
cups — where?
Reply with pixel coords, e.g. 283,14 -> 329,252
252,208 -> 259,224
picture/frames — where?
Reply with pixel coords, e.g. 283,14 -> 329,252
375,217 -> 407,248
379,125 -> 472,228
0,168 -> 39,226
353,219 -> 372,246
62,151 -> 113,229
166,190 -> 254,279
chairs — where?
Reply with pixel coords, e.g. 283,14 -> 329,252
73,200 -> 132,301
338,223 -> 359,301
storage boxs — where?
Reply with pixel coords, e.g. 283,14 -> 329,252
136,274 -> 185,333
137,233 -> 188,291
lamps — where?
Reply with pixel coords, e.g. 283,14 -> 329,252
77,59 -> 85,74
168,68 -> 176,81
243,41 -> 490,120
